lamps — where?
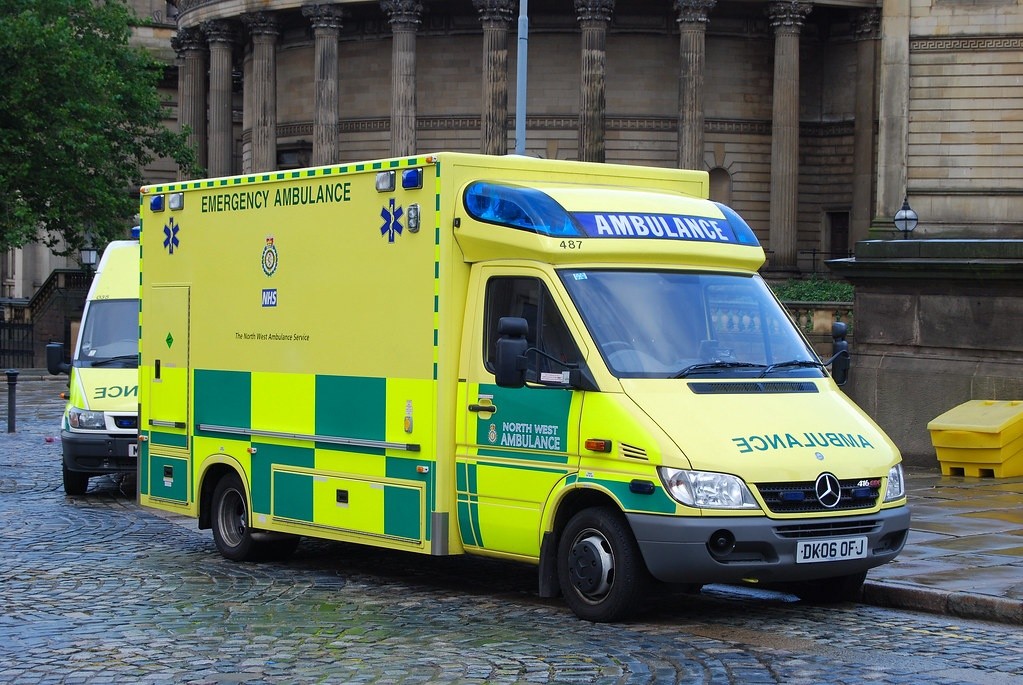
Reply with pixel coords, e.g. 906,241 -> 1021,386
894,197 -> 920,239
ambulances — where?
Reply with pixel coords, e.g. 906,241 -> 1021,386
45,223 -> 144,502
138,151 -> 912,624
